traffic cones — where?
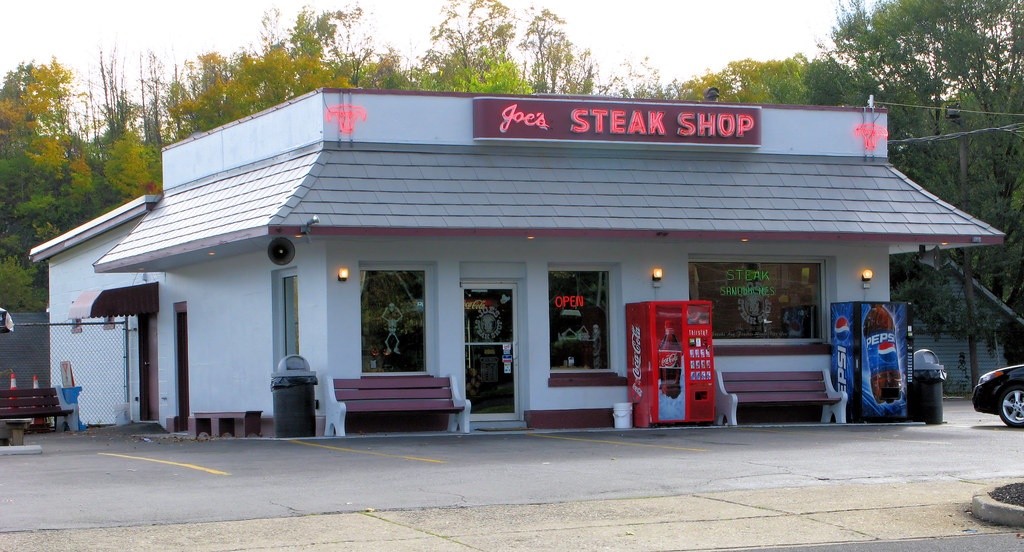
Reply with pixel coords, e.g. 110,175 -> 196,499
7,373 -> 20,410
30,375 -> 51,434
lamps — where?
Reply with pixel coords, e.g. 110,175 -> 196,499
651,268 -> 663,281
338,268 -> 349,281
300,215 -> 320,232
862,269 -> 873,282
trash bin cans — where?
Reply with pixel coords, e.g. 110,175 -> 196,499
613,402 -> 633,428
907,349 -> 947,424
270,354 -> 319,438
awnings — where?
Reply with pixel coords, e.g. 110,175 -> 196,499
67,281 -> 160,319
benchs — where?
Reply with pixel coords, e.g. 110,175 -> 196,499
192,409 -> 263,438
324,373 -> 472,436
716,368 -> 848,424
0,387 -> 75,432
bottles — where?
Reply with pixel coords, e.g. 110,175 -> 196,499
658,319 -> 682,398
863,303 -> 902,404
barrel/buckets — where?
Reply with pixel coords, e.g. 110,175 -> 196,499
613,403 -> 633,428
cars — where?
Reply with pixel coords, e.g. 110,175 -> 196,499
972,363 -> 1024,428
